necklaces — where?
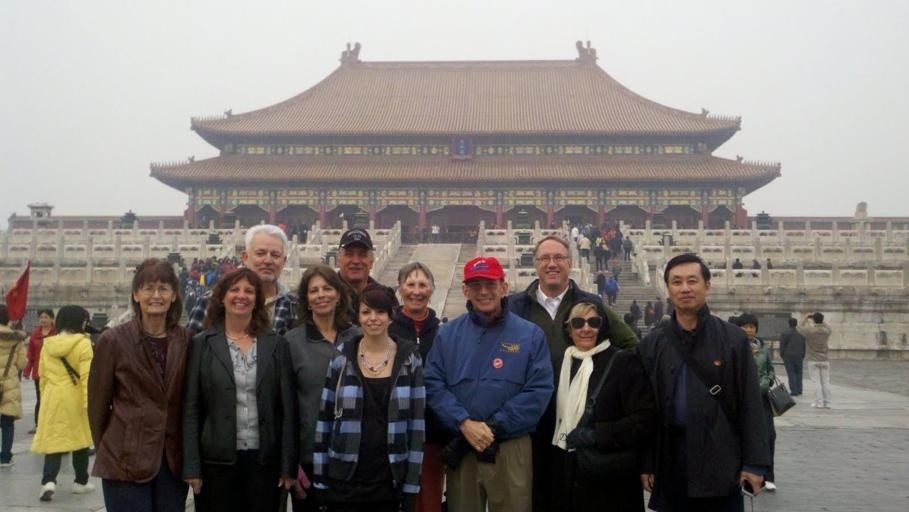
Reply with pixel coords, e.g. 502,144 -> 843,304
224,328 -> 250,342
359,334 -> 393,375
142,326 -> 171,338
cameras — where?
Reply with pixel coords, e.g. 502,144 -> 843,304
808,314 -> 815,319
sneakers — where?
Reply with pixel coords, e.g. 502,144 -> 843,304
72,482 -> 95,495
38,481 -> 55,501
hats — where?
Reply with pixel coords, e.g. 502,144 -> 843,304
339,228 -> 373,251
536,255 -> 568,264
461,256 -> 505,284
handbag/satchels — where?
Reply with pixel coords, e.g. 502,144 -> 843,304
549,350 -> 623,477
767,372 -> 796,418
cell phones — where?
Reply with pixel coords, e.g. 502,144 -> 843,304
740,480 -> 754,497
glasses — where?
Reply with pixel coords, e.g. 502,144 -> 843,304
143,284 -> 172,295
570,316 -> 602,330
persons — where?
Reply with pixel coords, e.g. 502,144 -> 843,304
633,253 -> 774,511
328,227 -> 396,328
412,224 -> 421,242
734,313 -> 779,493
504,236 -> 639,360
549,219 -> 555,231
749,257 -> 761,278
174,255 -> 238,314
530,295 -> 646,512
184,268 -> 293,511
182,220 -> 306,337
421,224 -> 429,242
766,258 -> 773,270
277,219 -> 308,244
21,309 -> 58,437
422,256 -> 555,512
557,222 -> 561,230
0,305 -> 27,465
569,217 -> 669,336
389,262 -> 443,511
35,306 -> 94,500
275,263 -> 363,512
313,286 -> 425,512
731,257 -> 744,278
87,257 -> 193,512
430,223 -> 441,242
708,262 -> 713,269
778,317 -> 806,395
796,311 -> 832,409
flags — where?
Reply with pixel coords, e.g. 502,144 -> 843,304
5,262 -> 31,320
